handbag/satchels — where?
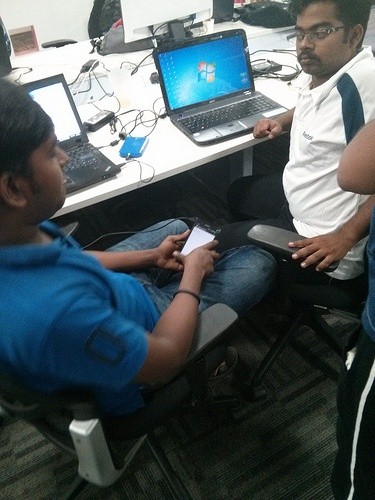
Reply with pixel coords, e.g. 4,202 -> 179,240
97,18 -> 155,56
231,0 -> 296,28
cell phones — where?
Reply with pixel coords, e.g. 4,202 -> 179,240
180,220 -> 221,257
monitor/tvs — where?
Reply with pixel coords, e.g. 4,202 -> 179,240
118,0 -> 212,44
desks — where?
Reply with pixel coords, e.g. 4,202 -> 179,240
1,5 -> 374,218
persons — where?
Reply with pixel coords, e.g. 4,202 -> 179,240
331,120 -> 375,500
0,79 -> 276,418
254,0 -> 375,313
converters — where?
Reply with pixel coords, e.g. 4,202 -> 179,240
82,60 -> 99,72
251,61 -> 282,76
83,110 -> 114,132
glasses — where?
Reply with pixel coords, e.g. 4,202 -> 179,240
287,24 -> 355,43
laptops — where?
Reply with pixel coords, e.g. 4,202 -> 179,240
153,29 -> 290,146
19,73 -> 121,194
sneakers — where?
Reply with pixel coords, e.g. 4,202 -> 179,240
208,346 -> 238,380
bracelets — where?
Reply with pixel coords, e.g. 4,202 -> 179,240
173,287 -> 203,305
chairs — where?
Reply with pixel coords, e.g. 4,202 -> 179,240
0,302 -> 260,499
241,222 -> 368,401
